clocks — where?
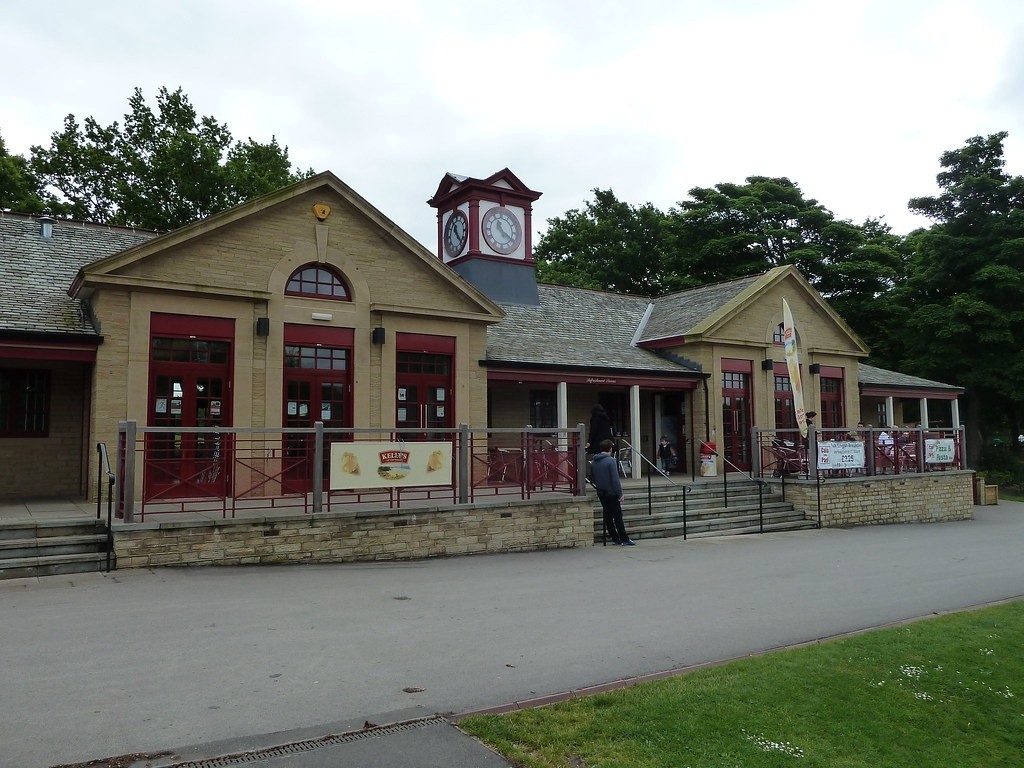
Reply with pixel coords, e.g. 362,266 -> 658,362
444,210 -> 469,257
482,207 -> 523,255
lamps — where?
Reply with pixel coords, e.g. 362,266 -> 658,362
373,327 -> 385,345
257,317 -> 269,337
809,363 -> 820,374
761,359 -> 773,370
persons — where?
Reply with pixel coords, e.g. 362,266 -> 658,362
586,404 -> 612,460
592,440 -> 637,546
657,437 -> 676,475
806,411 -> 893,444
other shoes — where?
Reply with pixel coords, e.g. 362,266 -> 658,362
665,471 -> 670,475
612,540 -> 621,545
882,471 -> 889,475
662,469 -> 665,474
621,539 -> 635,545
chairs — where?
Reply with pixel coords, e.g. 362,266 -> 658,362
497,447 -> 633,482
765,431 -> 947,477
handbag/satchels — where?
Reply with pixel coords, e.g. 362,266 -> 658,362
700,442 -> 717,455
671,456 -> 678,465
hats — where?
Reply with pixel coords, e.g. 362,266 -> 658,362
599,439 -> 614,450
